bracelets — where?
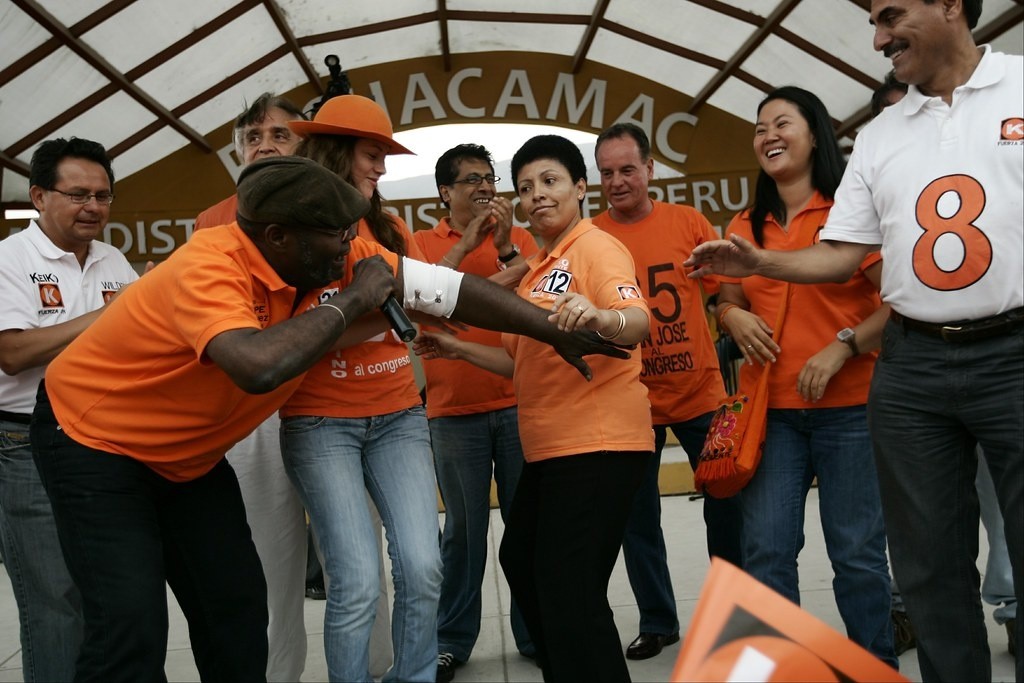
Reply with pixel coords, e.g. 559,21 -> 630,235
596,309 -> 626,341
443,255 -> 459,270
720,304 -> 740,333
319,303 -> 347,330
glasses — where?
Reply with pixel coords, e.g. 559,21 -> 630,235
446,173 -> 501,188
38,182 -> 117,206
279,215 -> 353,242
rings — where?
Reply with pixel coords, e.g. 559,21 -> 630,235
577,304 -> 584,313
746,344 -> 753,350
431,346 -> 436,351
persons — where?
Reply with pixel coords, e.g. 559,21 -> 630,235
29,154 -> 639,683
193,90 -> 470,683
1,133 -> 155,683
684,0 -> 1024,683
412,123 -> 724,683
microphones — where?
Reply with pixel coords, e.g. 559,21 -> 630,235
354,259 -> 417,342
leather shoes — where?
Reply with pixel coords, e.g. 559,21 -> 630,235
303,583 -> 326,599
626,630 -> 681,659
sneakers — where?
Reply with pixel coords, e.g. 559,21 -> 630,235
891,607 -> 917,656
432,647 -> 468,683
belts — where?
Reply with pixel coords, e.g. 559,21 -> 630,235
887,305 -> 1024,346
0,408 -> 32,424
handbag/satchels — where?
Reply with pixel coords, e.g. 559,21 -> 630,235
697,379 -> 770,496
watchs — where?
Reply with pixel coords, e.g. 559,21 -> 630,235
836,327 -> 860,356
498,243 -> 521,263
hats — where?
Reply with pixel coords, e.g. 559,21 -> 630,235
287,94 -> 418,156
236,154 -> 371,231
